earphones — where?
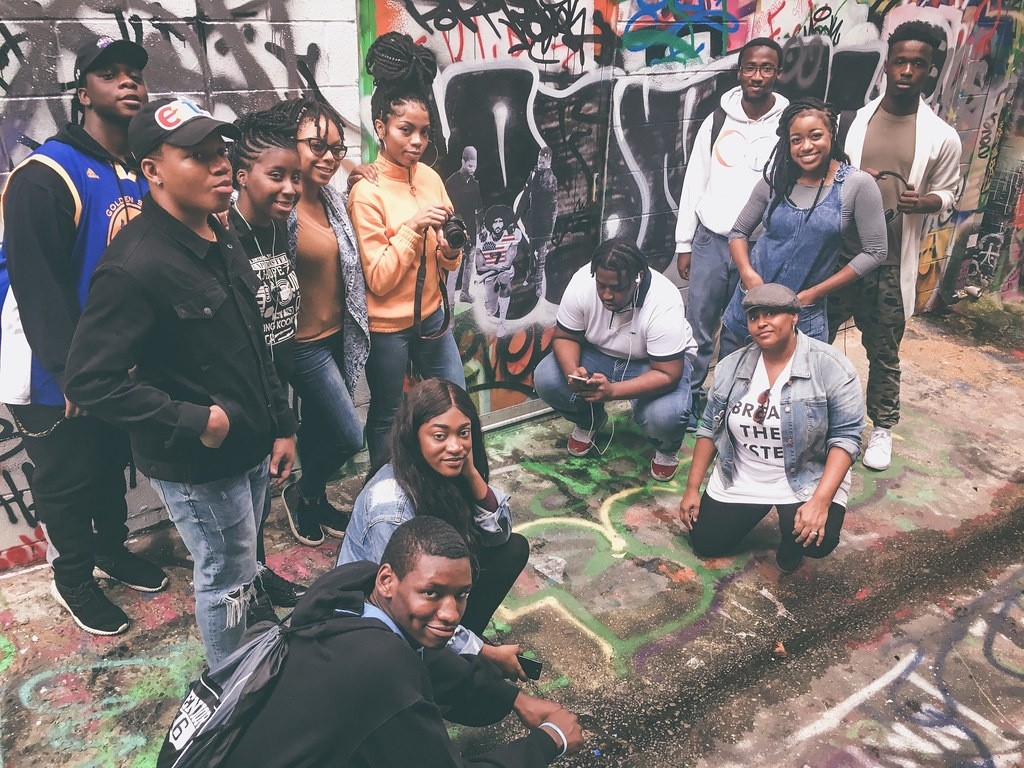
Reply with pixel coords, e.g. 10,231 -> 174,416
636,273 -> 641,282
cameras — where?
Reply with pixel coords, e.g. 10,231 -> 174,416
431,213 -> 467,249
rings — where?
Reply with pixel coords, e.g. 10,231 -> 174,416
810,531 -> 817,535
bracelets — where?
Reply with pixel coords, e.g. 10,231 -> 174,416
538,721 -> 567,762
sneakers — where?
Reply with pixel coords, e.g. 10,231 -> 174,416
651,445 -> 683,481
776,541 -> 803,572
255,567 -> 310,608
281,483 -> 325,546
316,493 -> 351,538
92,546 -> 169,592
50,576 -> 129,635
686,394 -> 700,431
569,412 -> 608,456
862,427 -> 894,470
246,594 -> 283,630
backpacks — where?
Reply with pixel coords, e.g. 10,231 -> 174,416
155,613 -> 390,768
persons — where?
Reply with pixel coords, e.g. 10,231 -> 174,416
831,21 -> 962,471
446,145 -> 557,338
1,35 -> 168,636
679,283 -> 866,575
334,377 -> 528,684
717,95 -> 888,365
223,514 -> 583,768
533,238 -> 698,480
221,114 -> 310,630
671,36 -> 789,431
210,99 -> 380,546
349,81 -> 466,478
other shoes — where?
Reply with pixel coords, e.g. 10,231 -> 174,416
460,290 -> 476,303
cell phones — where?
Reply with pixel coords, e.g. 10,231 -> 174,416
516,655 -> 542,680
569,374 -> 592,387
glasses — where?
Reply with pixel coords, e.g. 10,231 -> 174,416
737,62 -> 782,79
297,135 -> 348,162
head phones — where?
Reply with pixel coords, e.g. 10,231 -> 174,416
874,171 -> 910,223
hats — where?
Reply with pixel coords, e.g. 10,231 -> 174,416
740,282 -> 802,315
127,97 -> 241,162
74,35 -> 149,84
